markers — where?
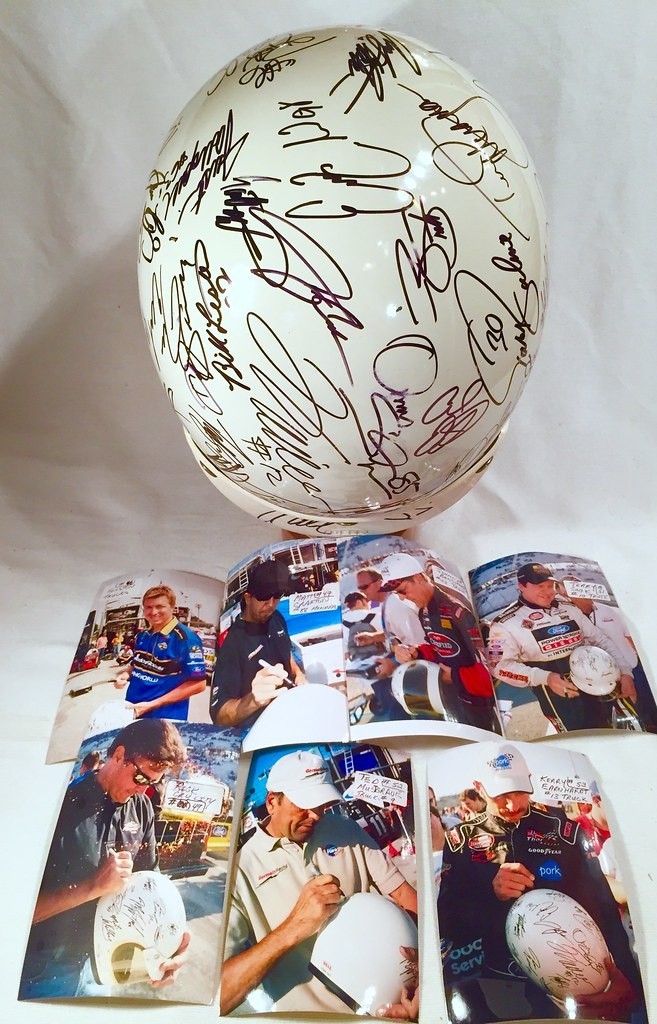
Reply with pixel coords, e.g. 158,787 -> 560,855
356,632 -> 358,647
258,659 -> 295,687
394,637 -> 416,659
311,868 -> 346,898
106,845 -> 116,856
107,679 -> 117,682
559,672 -> 568,698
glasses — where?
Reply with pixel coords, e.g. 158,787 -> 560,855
129,754 -> 158,786
250,592 -> 285,601
357,580 -> 376,591
398,579 -> 412,595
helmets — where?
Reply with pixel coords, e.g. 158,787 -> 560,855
391,659 -> 448,716
241,684 -> 350,753
90,868 -> 186,987
308,890 -> 419,1017
569,645 -> 622,702
506,887 -> 615,1017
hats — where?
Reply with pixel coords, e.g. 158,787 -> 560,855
246,560 -> 291,596
473,743 -> 534,798
125,646 -> 130,651
378,553 -> 424,592
267,750 -> 345,810
518,562 -> 561,584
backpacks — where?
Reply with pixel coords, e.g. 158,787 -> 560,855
344,613 -> 387,662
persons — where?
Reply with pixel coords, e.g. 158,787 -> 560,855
210,543 -> 339,730
481,562 -> 657,734
429,744 -> 649,1024
219,749 -> 419,1024
77,585 -> 218,721
18,721 -> 233,1000
343,540 -> 502,736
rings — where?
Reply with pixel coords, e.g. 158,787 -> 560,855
601,1017 -> 605,1020
